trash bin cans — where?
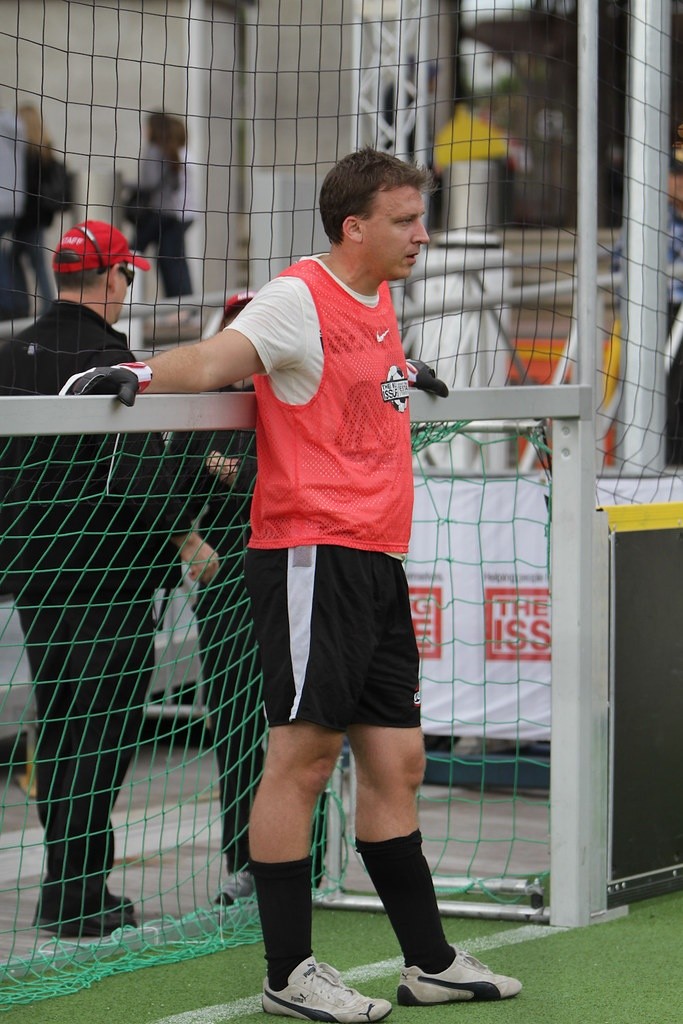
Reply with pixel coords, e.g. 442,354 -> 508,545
440,157 -> 502,232
69,169 -> 124,237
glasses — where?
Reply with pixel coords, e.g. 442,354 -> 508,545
119,264 -> 135,286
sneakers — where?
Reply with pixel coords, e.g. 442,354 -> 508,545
398,943 -> 523,1007
215,871 -> 252,904
263,957 -> 392,1024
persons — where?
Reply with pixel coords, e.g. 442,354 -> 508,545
114,107 -> 197,294
168,291 -> 263,905
0,101 -> 73,318
610,126 -> 682,458
56,150 -> 520,1023
433,92 -> 535,183
383,55 -> 440,150
0,219 -> 219,934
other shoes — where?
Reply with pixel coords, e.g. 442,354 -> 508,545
31,894 -> 137,937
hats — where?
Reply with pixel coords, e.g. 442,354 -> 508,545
220,289 -> 256,324
53,222 -> 151,272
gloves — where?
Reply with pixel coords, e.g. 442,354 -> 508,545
58,362 -> 153,407
404,358 -> 449,398
